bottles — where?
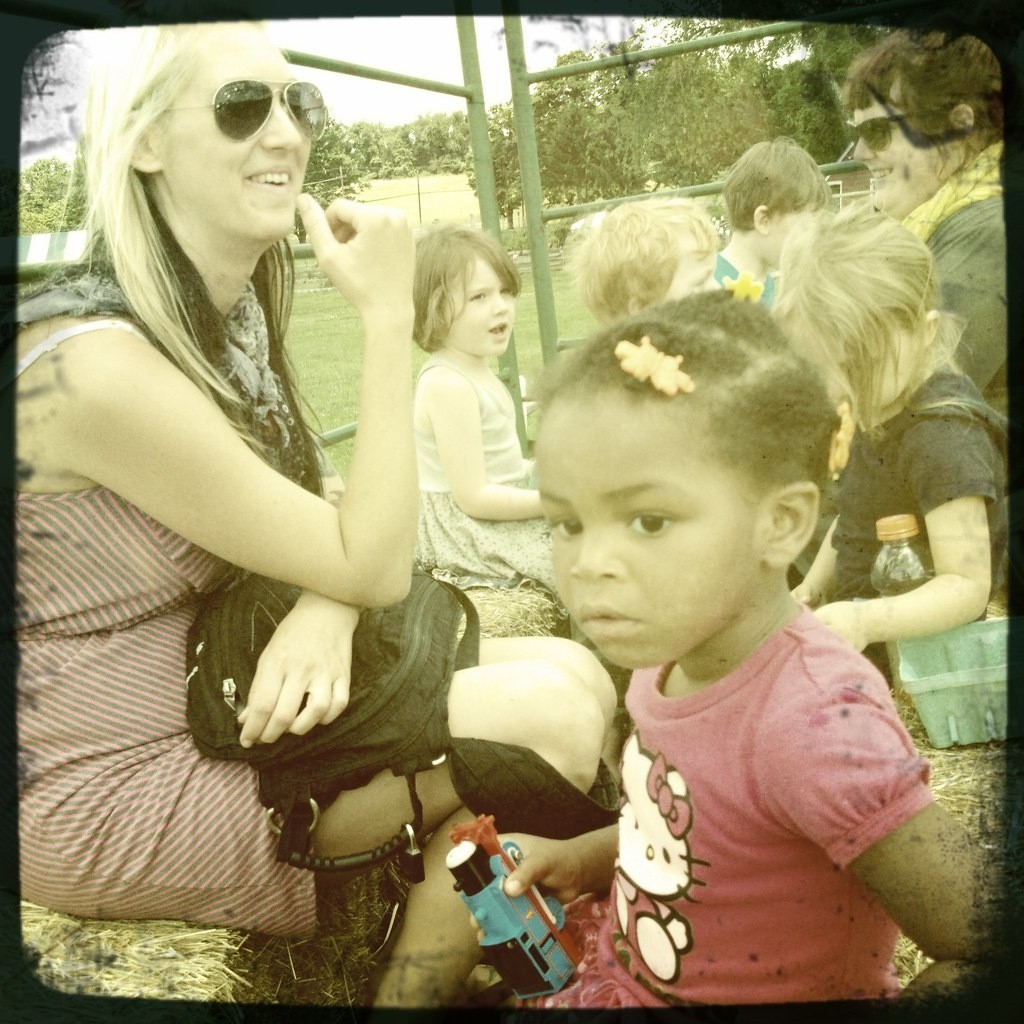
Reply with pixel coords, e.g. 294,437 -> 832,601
869,513 -> 936,696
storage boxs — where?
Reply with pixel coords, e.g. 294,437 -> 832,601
896,616 -> 1009,752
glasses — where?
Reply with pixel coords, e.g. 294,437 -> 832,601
163,81 -> 326,140
845,112 -> 915,151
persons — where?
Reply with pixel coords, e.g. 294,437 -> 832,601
579,197 -> 720,327
452,287 -> 1000,1008
786,31 -> 1008,690
768,211 -> 1010,685
15,19 -> 617,1006
718,139 -> 834,316
411,223 -> 634,696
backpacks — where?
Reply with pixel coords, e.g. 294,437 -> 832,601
188,571 -> 621,873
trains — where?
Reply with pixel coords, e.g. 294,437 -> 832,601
444,814 -> 581,1001
